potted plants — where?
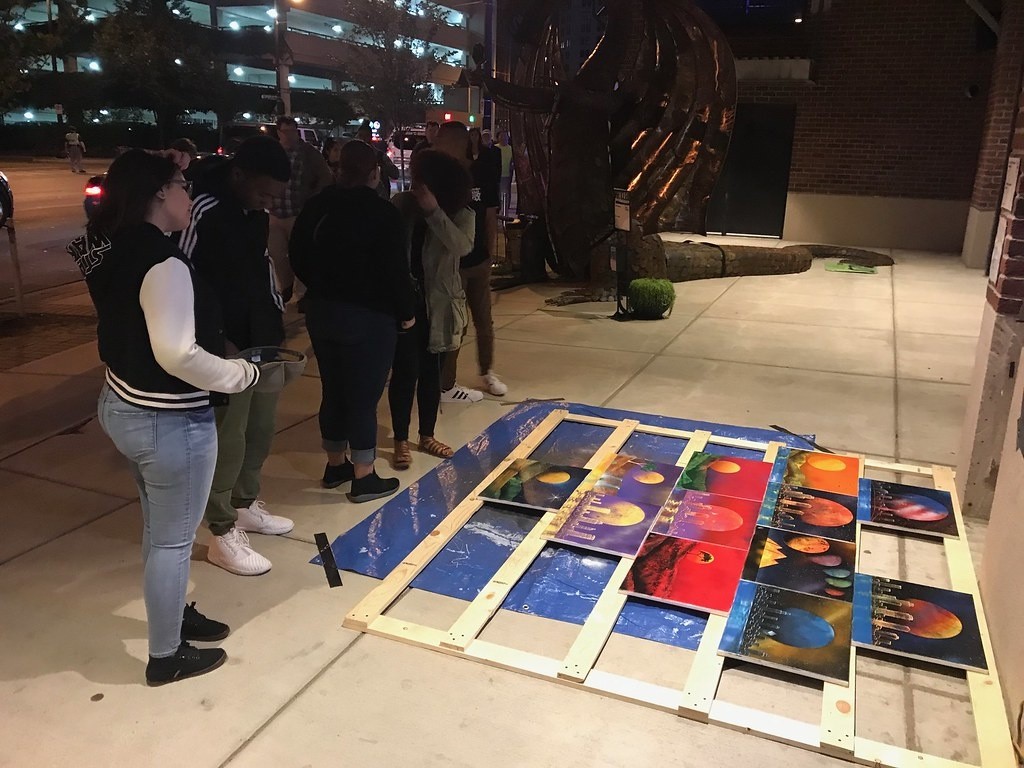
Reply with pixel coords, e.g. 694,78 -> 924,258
626,278 -> 676,321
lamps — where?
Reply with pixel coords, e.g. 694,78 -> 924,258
791,8 -> 808,25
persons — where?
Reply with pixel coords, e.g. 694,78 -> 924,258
65,126 -> 86,172
97,116 -> 512,686
390,149 -> 475,467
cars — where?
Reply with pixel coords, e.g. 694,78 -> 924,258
81,171 -> 112,223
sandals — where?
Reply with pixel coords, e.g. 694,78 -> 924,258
418,434 -> 454,458
393,438 -> 411,469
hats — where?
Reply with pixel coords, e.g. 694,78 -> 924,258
227,345 -> 307,393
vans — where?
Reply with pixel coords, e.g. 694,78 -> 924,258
219,122 -> 325,162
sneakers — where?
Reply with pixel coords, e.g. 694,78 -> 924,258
207,528 -> 273,577
480,369 -> 508,396
146,640 -> 226,685
350,469 -> 400,502
323,456 -> 354,488
182,601 -> 230,641
235,500 -> 294,535
440,381 -> 483,403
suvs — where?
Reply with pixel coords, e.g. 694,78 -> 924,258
383,125 -> 427,191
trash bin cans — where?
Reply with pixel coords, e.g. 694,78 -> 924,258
504,218 -> 533,270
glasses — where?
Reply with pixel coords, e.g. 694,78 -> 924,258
167,179 -> 193,197
368,165 -> 381,175
